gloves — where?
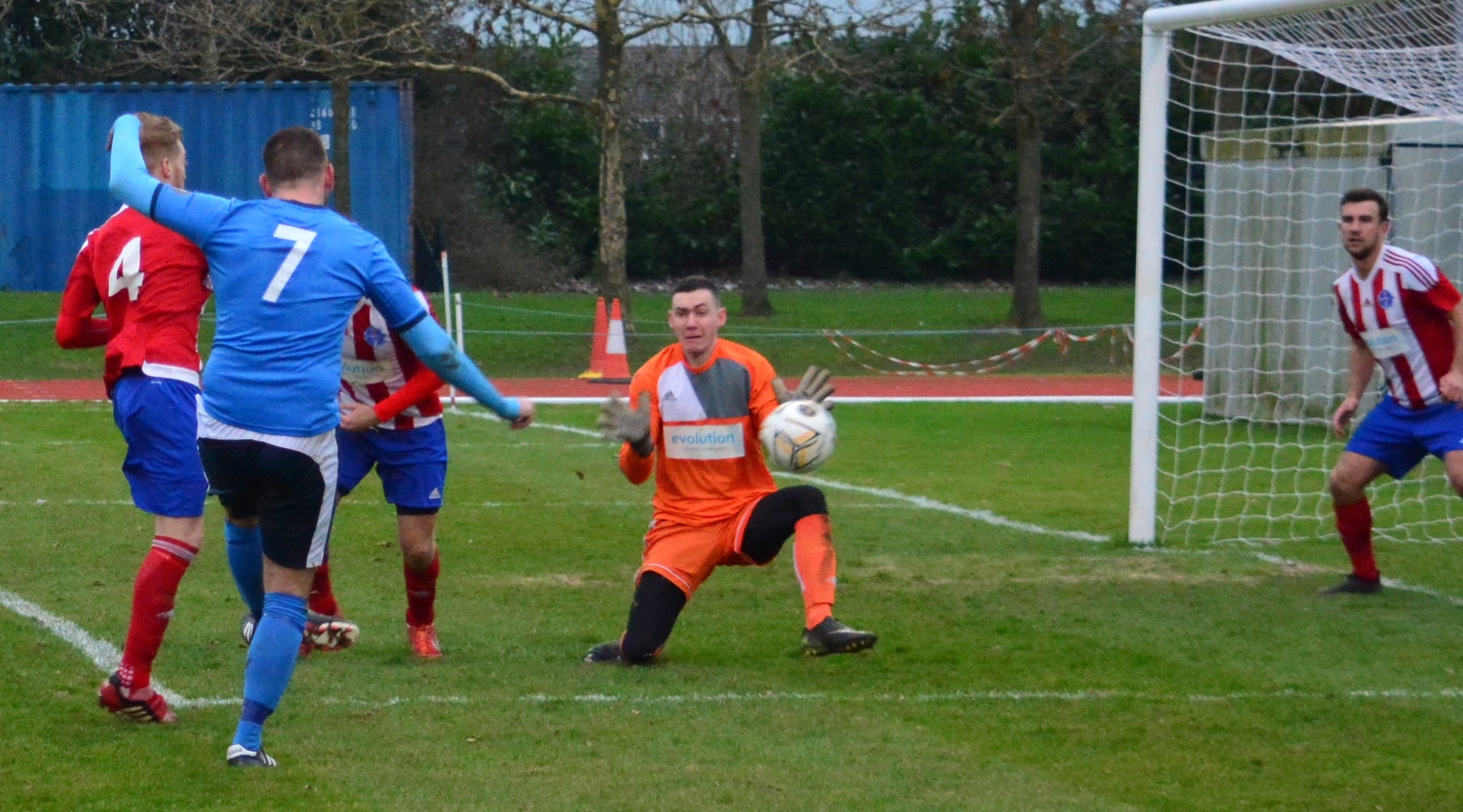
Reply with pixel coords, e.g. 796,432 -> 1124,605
595,388 -> 654,458
773,365 -> 838,412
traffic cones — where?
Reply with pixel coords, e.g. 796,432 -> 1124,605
579,293 -> 609,376
590,296 -> 632,383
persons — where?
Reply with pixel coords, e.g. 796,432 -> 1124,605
55,114 -> 360,724
1316,185 -> 1463,599
295,281 -> 448,659
107,113 -> 535,768
583,275 -> 879,668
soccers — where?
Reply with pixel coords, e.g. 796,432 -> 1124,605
758,399 -> 838,474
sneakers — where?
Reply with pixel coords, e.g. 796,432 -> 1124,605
800,615 -> 878,658
404,613 -> 443,663
585,640 -> 661,666
242,614 -> 257,644
299,607 -> 360,659
1319,572 -> 1383,594
227,745 -> 280,769
97,669 -> 180,726
